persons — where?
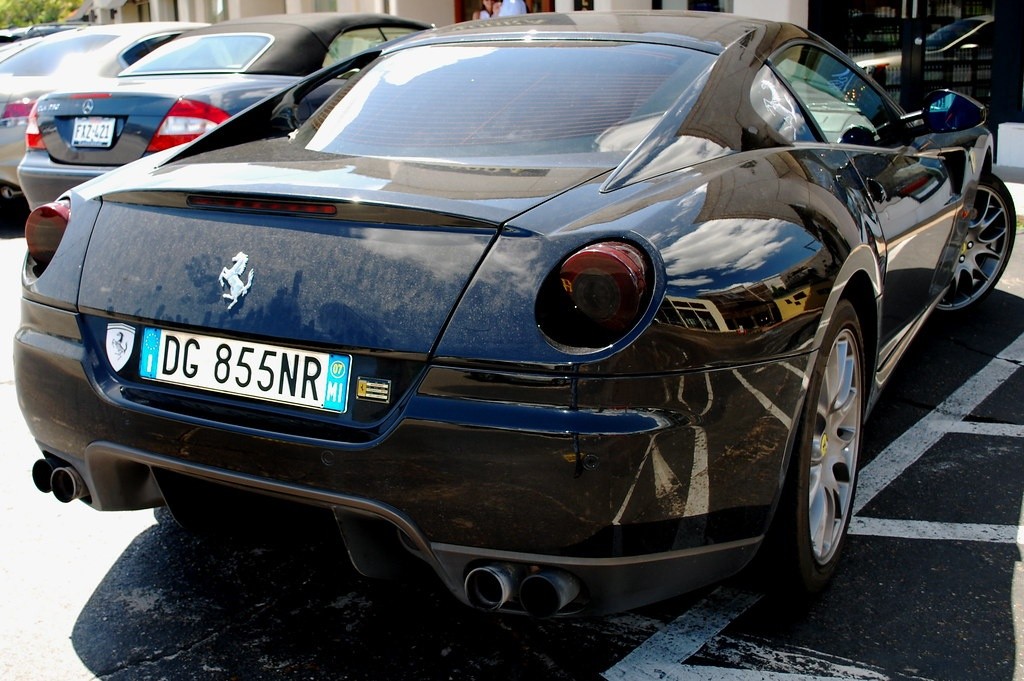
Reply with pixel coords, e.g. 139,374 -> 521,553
492,2 -> 501,17
498,0 -> 527,17
472,0 -> 498,20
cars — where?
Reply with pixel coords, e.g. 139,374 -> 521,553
850,15 -> 993,108
16,11 -> 437,208
0,21 -> 215,205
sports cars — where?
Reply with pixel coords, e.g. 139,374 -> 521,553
9,8 -> 1016,617
